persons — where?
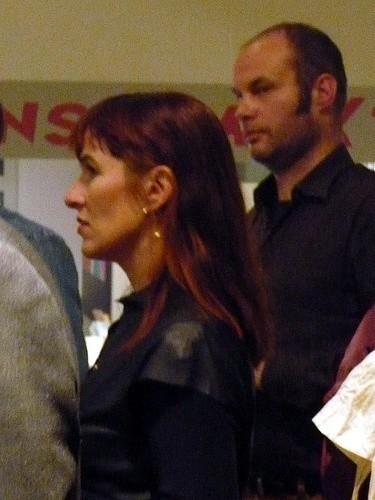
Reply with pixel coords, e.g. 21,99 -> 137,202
0,208 -> 90,500
312,304 -> 375,500
231,23 -> 375,500
66,91 -> 275,500
